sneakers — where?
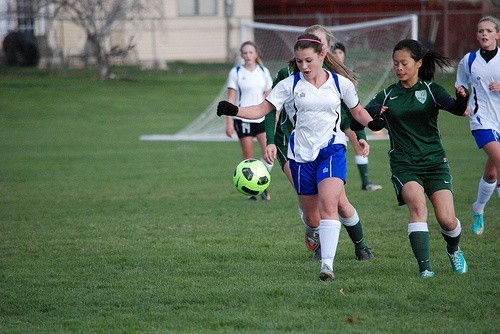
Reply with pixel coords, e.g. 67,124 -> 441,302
248,196 -> 257,200
356,246 -> 377,260
445,245 -> 468,275
419,270 -> 434,280
262,189 -> 271,200
305,228 -> 321,262
471,201 -> 484,237
320,264 -> 335,281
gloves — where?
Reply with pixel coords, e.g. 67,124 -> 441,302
217,101 -> 238,116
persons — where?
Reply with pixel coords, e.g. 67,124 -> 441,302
350,39 -> 470,278
217,25 -> 384,280
455,16 -> 500,236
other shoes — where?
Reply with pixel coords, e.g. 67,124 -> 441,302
361,184 -> 382,192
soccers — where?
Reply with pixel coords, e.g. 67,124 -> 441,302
232,158 -> 272,197
